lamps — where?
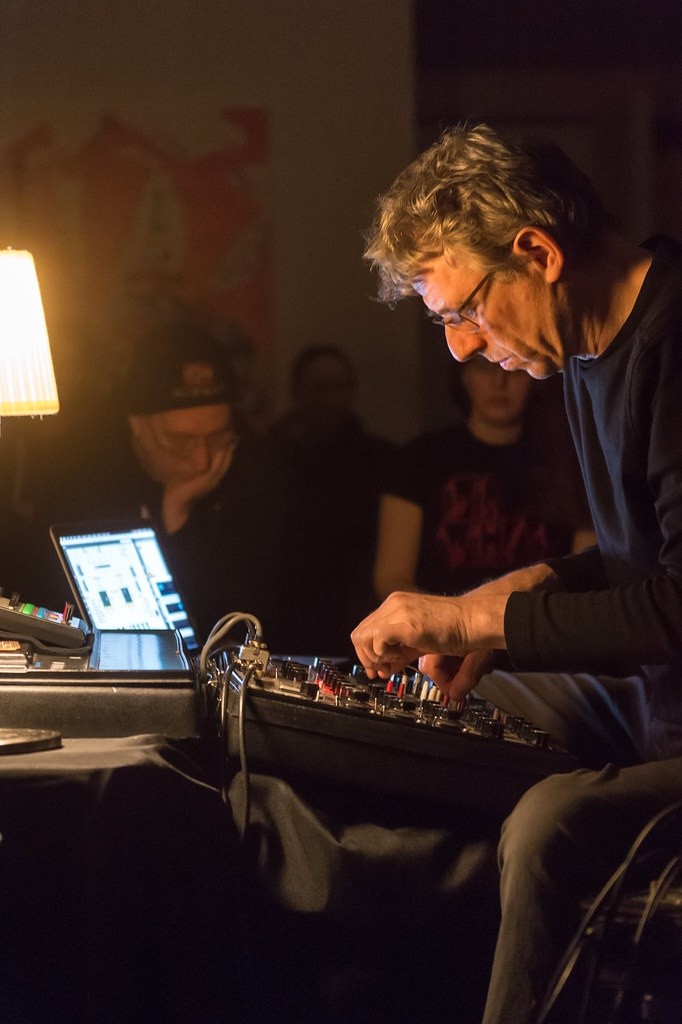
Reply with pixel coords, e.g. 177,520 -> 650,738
0,246 -> 60,417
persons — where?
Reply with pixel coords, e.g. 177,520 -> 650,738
23,318 -> 598,645
348,124 -> 682,1024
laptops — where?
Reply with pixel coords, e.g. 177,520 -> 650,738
52,518 -> 204,664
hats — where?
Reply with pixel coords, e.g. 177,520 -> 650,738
123,321 -> 235,415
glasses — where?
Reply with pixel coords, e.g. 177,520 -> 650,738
432,270 -> 496,335
149,415 -> 240,456
